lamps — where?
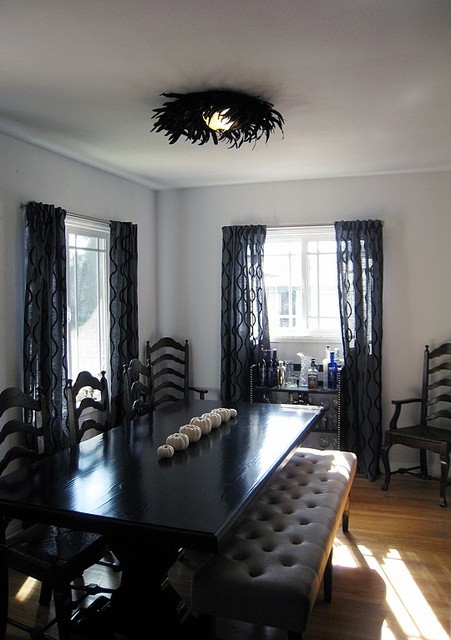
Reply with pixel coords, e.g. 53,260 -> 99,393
150,88 -> 285,152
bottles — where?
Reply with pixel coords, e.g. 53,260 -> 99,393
257,343 -> 286,388
328,351 -> 338,391
322,345 -> 331,390
287,392 -> 312,405
308,359 -> 318,390
334,348 -> 343,369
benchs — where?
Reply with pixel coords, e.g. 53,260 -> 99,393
210,446 -> 359,638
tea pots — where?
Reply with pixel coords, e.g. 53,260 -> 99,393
297,353 -> 320,387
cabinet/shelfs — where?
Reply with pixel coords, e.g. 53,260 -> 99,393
250,362 -> 346,451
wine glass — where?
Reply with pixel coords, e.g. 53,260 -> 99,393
319,433 -> 337,450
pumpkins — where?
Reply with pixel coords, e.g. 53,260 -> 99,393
156,445 -> 175,458
201,412 -> 222,428
165,433 -> 190,450
189,417 -> 212,435
228,409 -> 237,416
178,424 -> 202,442
212,408 -> 231,422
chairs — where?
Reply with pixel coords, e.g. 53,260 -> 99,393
379,341 -> 451,508
65,372 -> 113,445
0,385 -> 112,635
121,355 -> 154,420
143,337 -> 208,406
161,549 -> 165,553
